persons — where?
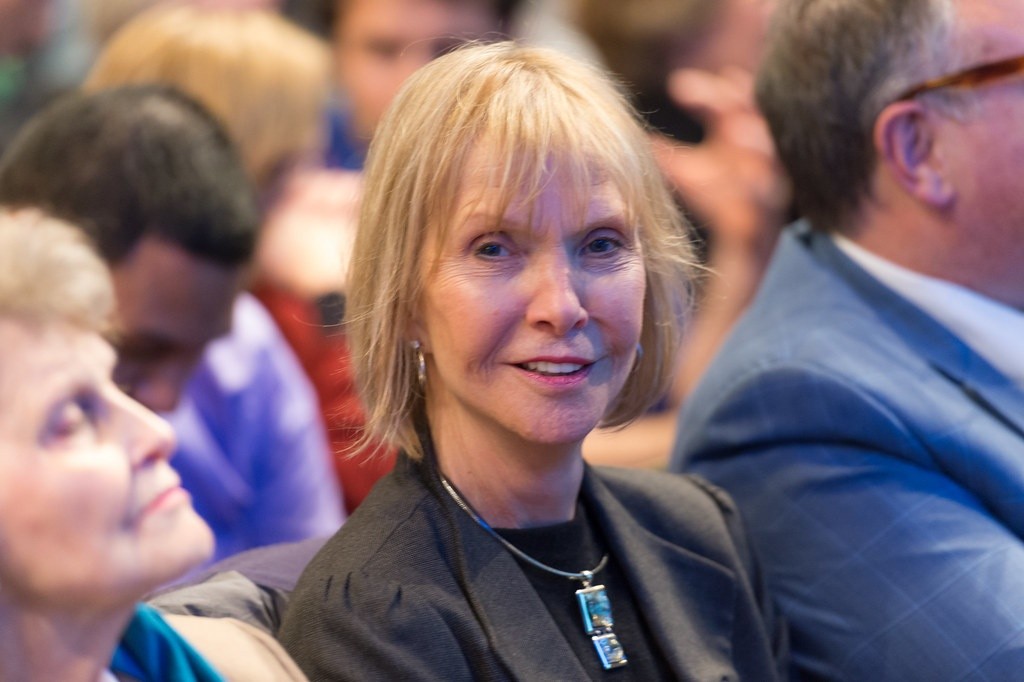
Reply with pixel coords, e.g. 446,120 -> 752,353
0,0 -> 1024,682
276,36 -> 789,682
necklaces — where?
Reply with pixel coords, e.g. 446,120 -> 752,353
440,474 -> 628,670
